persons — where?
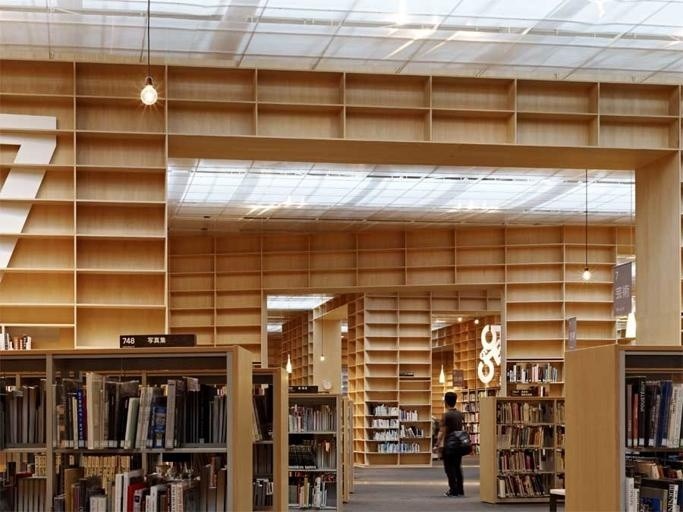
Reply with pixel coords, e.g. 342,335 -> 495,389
430,390 -> 469,497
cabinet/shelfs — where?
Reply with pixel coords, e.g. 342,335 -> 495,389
561,340 -> 682,512
474,392 -> 565,505
1,56 -> 167,348
0,342 -> 354,512
169,220 -> 636,472
168,65 -> 682,148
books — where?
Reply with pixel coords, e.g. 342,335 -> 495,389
1,372 -> 337,511
366,390 -> 498,456
5,333 -> 32,350
399,370 -> 414,376
495,358 -> 683,511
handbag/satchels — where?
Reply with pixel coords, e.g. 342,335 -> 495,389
445,430 -> 472,449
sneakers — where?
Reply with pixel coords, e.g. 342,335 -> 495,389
444,487 -> 465,497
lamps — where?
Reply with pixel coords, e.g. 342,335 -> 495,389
140,0 -> 158,106
